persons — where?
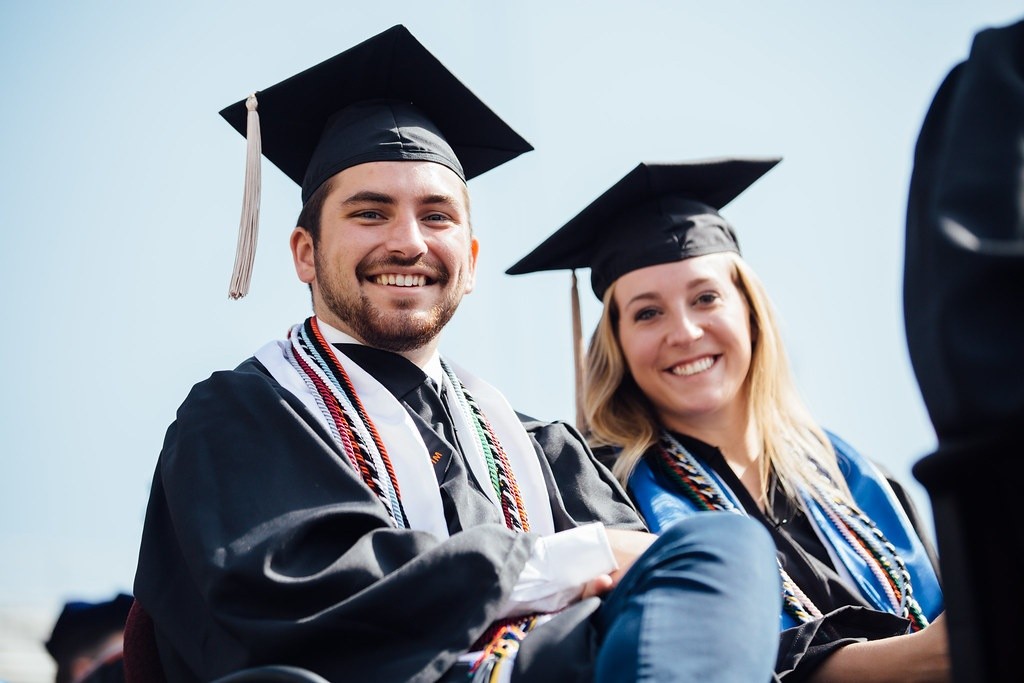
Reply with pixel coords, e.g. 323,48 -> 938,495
504,151 -> 950,683
43,592 -> 140,683
123,22 -> 786,683
903,13 -> 1024,683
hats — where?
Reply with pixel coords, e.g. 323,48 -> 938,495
217,24 -> 536,300
505,157 -> 783,436
